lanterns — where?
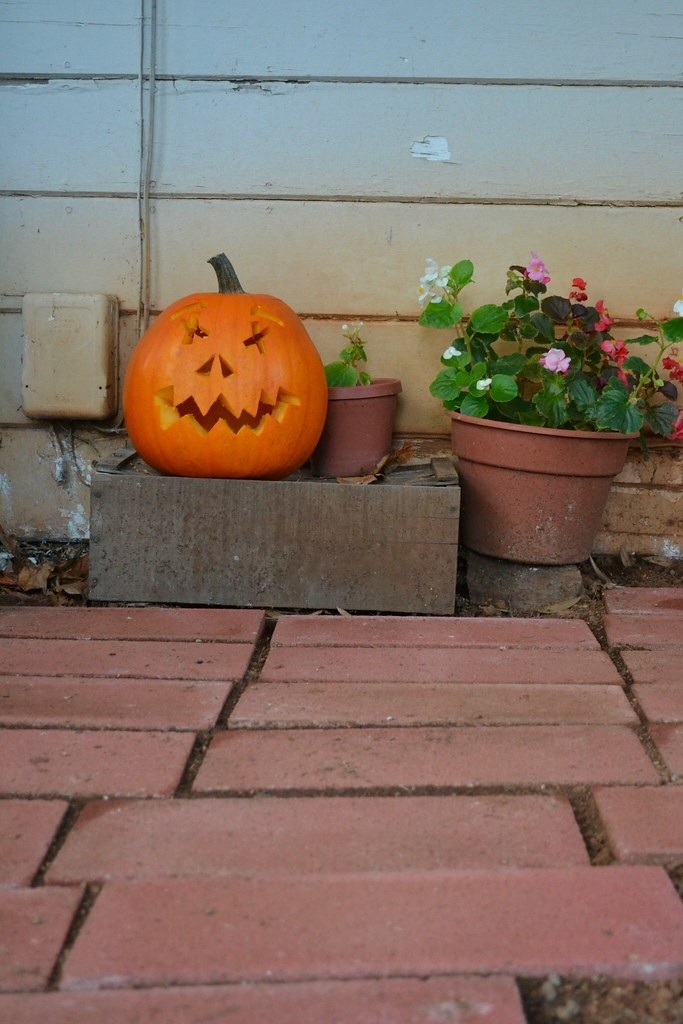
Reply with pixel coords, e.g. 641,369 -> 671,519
121,250 -> 330,482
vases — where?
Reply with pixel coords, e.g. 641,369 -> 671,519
298,379 -> 394,478
444,409 -> 638,564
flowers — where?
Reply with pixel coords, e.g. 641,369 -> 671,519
412,249 -> 683,443
317,319 -> 372,388
